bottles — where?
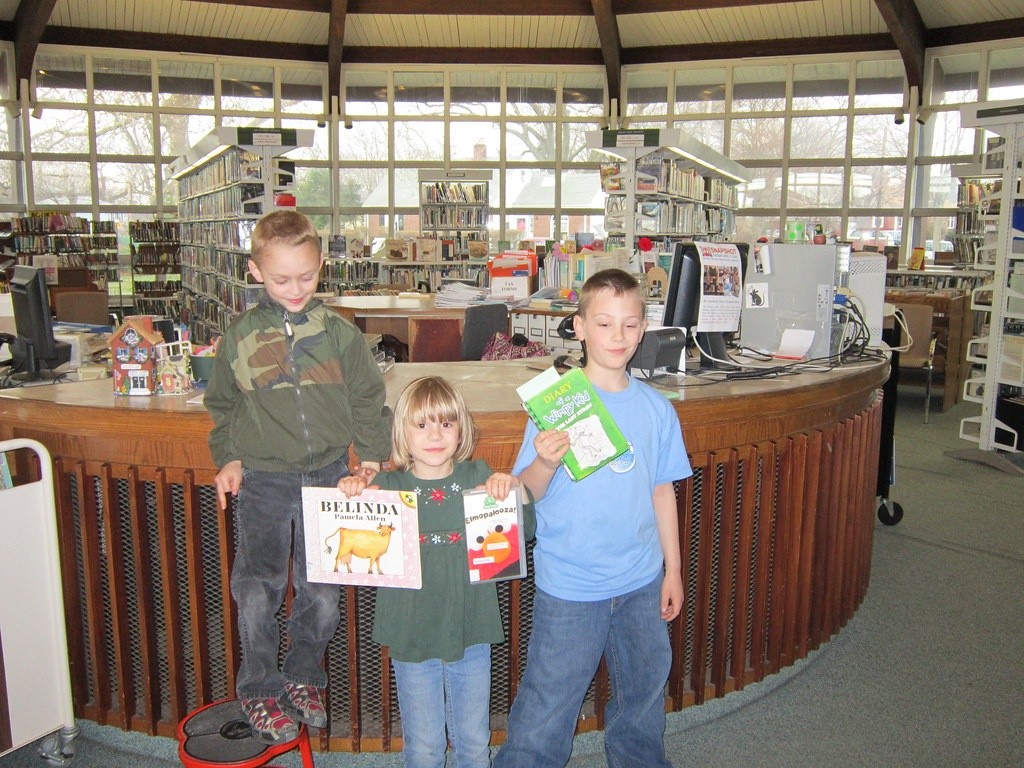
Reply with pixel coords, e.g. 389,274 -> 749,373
814,224 -> 826,245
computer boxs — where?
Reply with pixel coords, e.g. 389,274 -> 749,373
739,242 -> 851,367
843,251 -> 887,346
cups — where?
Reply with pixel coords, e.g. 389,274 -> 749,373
632,273 -> 650,301
191,356 -> 217,381
786,221 -> 807,244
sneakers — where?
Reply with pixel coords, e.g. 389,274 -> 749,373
281,681 -> 327,728
241,696 -> 299,745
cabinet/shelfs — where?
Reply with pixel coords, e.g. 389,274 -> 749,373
943,123 -> 1024,476
0,170 -> 494,346
587,126 -> 754,305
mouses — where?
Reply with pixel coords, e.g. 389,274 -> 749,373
554,355 -> 583,369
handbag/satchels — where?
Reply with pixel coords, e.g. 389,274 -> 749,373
481,333 -> 547,362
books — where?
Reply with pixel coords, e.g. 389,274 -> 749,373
529,156 -> 737,311
522,367 -> 629,482
275,155 -> 489,297
461,485 -> 528,584
301,486 -> 421,590
864,138 -> 1024,452
11,147 -> 264,349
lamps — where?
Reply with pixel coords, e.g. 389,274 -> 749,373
894,107 -> 904,124
32,106 -> 42,118
317,114 -> 326,127
7,103 -> 19,117
621,116 -> 631,130
344,117 -> 352,129
917,107 -> 934,124
599,118 -> 608,128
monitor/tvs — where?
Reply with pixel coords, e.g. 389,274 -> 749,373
6,262 -> 67,381
660,242 -> 750,372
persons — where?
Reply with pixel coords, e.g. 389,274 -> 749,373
490,268 -> 694,768
338,375 -> 538,768
205,210 -> 394,746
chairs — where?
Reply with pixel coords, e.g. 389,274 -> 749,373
462,304 -> 509,362
886,302 -> 939,424
51,291 -> 120,328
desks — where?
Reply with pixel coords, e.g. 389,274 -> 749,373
510,301 -> 581,319
326,294 -> 467,363
884,294 -> 971,414
